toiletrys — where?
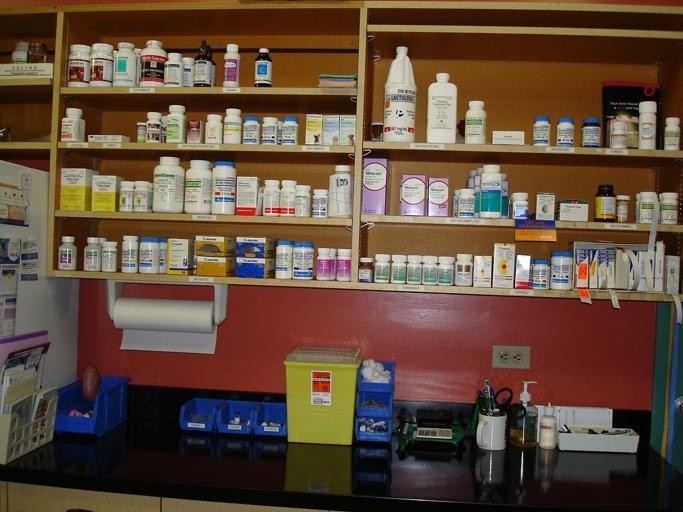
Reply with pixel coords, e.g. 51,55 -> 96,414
510,380 -> 538,446
509,447 -> 535,504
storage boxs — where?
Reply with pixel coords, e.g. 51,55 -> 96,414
281,347 -> 363,446
283,444 -> 352,497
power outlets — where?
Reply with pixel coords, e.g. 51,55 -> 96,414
491,345 -> 531,370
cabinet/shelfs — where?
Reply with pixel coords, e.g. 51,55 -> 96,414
0,0 -> 681,306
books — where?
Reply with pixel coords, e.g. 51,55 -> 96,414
0,328 -> 59,465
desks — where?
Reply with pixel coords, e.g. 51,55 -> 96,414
0,417 -> 682,512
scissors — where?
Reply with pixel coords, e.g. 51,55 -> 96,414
493,387 -> 526,421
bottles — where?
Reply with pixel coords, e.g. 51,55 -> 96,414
454,162 -> 527,219
595,185 -> 630,225
120,156 -> 352,219
666,118 -> 681,153
135,104 -> 300,148
65,39 -> 213,85
530,251 -> 575,291
223,40 -> 241,86
463,99 -> 487,146
532,116 -> 603,147
359,252 -> 474,289
275,238 -> 352,280
638,99 -> 659,150
58,232 -> 167,275
636,190 -> 677,225
539,451 -> 554,496
425,71 -> 457,143
252,46 -> 273,85
11,40 -> 48,63
62,106 -> 86,141
538,401 -> 560,450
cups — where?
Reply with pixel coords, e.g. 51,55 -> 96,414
476,451 -> 506,489
476,410 -> 508,450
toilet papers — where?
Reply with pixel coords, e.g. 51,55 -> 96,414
114,298 -> 218,357
